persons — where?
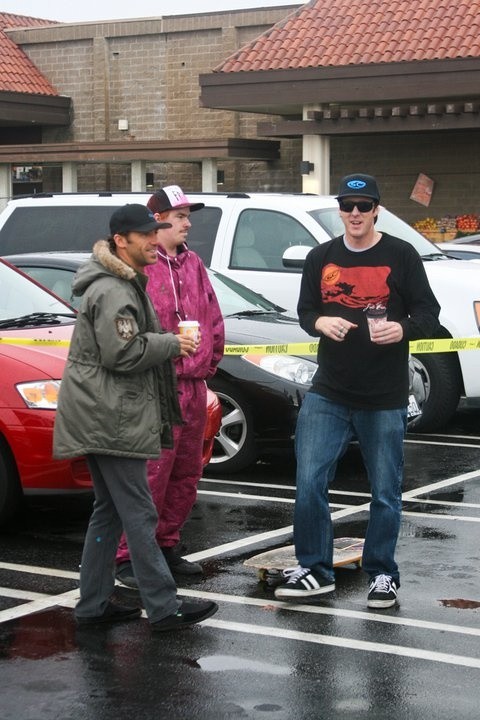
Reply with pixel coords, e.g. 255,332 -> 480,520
51,203 -> 220,631
273,173 -> 441,609
111,183 -> 224,588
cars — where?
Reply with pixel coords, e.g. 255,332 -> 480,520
0,255 -> 422,540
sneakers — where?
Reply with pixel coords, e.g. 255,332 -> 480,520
77,601 -> 142,626
151,602 -> 219,632
367,574 -> 398,607
275,567 -> 336,599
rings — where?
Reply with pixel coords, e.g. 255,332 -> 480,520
337,332 -> 341,337
339,327 -> 344,332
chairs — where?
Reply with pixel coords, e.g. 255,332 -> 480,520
230,223 -> 270,269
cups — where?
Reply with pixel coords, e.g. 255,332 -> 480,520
178,321 -> 199,343
366,307 -> 388,342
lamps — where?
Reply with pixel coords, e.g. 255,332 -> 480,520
216,170 -> 224,186
299,161 -> 315,176
145,172 -> 155,189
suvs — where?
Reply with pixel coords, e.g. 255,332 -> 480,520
1,191 -> 480,438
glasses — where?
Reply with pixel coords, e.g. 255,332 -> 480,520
337,198 -> 376,213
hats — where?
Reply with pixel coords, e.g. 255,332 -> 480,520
109,204 -> 172,234
335,174 -> 380,202
146,185 -> 205,215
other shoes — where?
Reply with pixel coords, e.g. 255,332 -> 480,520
162,546 -> 204,576
114,561 -> 137,590
